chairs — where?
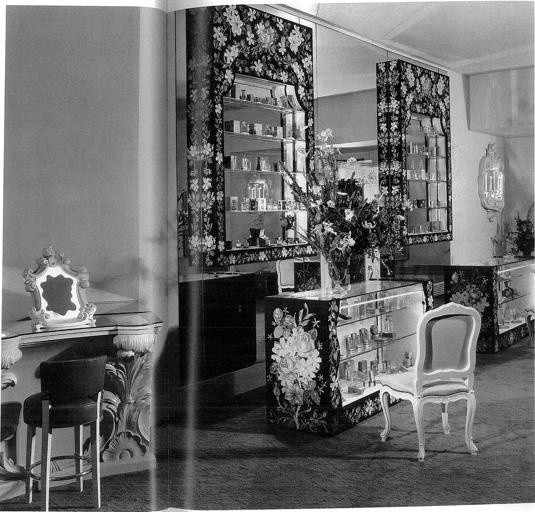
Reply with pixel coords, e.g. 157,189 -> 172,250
23,355 -> 107,510
374,302 -> 482,464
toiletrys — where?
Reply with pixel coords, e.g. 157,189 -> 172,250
338,290 -> 415,396
224,84 -> 302,251
407,127 -> 447,234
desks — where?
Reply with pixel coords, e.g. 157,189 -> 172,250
1,311 -> 164,503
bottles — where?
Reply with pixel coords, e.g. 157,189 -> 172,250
266,199 -> 293,210
236,240 -> 242,248
411,159 -> 417,179
249,183 -> 265,211
418,160 -> 426,181
240,198 -> 248,211
243,239 -> 250,248
276,237 -> 282,245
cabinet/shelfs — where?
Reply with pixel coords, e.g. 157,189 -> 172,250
408,253 -> 535,354
264,276 -> 434,437
174,1 -> 332,423
375,57 -> 454,310
